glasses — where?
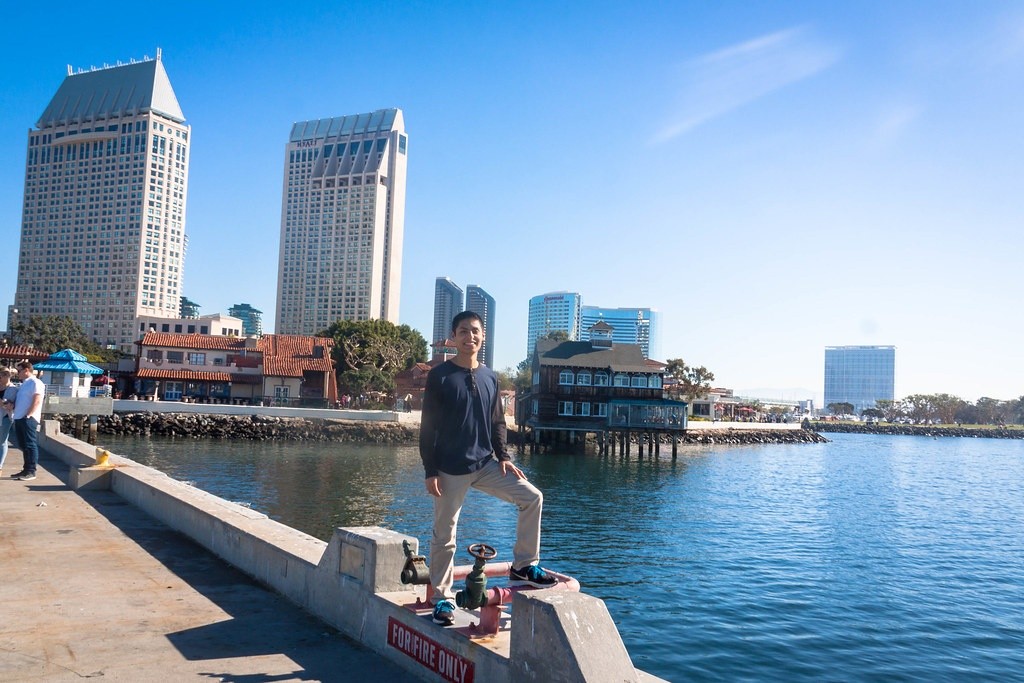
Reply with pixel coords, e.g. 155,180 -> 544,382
18,368 -> 25,373
470,373 -> 477,391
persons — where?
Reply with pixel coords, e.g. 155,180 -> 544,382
9,361 -> 44,481
416,312 -> 562,628
0,366 -> 18,476
333,390 -> 414,414
719,412 -> 812,425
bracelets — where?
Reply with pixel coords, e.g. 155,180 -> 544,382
25,415 -> 30,418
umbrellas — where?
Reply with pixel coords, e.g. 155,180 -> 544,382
95,375 -> 115,386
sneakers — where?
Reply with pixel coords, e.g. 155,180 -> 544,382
508,566 -> 559,589
432,605 -> 456,625
11,470 -> 36,480
